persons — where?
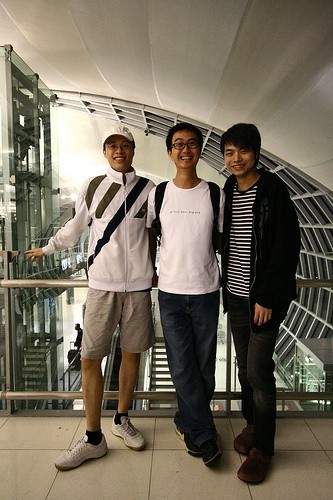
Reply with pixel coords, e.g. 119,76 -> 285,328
71,323 -> 83,371
24,126 -> 157,471
220,123 -> 301,482
146,123 -> 225,466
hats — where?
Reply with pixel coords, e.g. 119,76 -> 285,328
102,126 -> 134,150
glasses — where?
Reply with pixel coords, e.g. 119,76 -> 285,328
170,141 -> 201,150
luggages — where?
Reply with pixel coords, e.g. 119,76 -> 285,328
67,341 -> 79,368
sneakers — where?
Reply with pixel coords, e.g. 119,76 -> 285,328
201,436 -> 223,468
111,414 -> 146,451
54,434 -> 108,471
175,423 -> 200,457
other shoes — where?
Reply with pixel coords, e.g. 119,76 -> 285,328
237,447 -> 273,484
234,426 -> 252,455
